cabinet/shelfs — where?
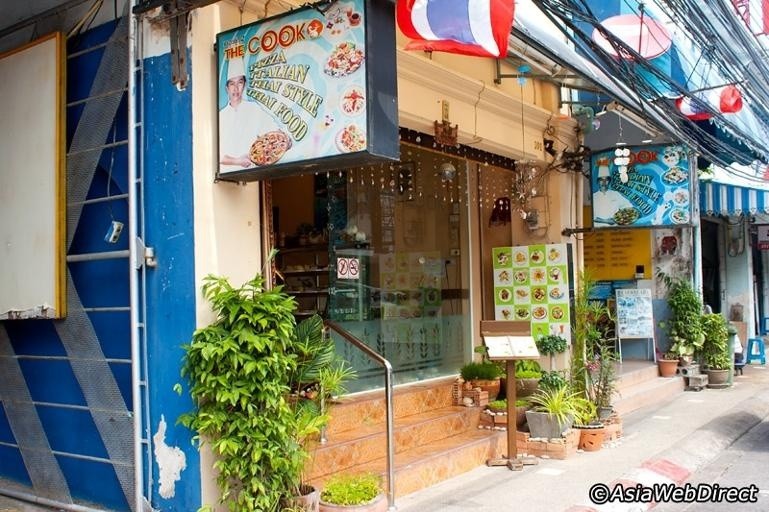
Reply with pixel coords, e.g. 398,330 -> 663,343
277,244 -> 363,317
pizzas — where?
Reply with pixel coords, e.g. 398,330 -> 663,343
340,86 -> 368,116
335,127 -> 366,154
613,207 -> 639,226
249,131 -> 289,166
325,42 -> 364,77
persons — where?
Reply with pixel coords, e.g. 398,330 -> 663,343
592,173 -> 632,226
217,74 -> 292,172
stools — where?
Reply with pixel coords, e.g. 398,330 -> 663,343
746,317 -> 769,365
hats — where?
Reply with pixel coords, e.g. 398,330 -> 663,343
226,42 -> 246,80
597,157 -> 611,178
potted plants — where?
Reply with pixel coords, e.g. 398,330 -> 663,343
181,273 -> 389,512
464,267 -> 618,452
656,283 -> 731,390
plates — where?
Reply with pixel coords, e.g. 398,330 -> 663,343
498,249 -> 564,321
